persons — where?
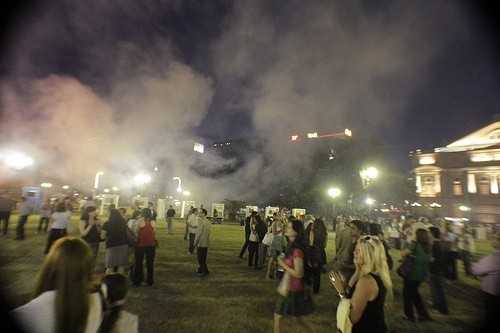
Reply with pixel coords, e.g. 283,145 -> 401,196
199,205 -> 203,211
165,205 -> 176,235
183,206 -> 195,240
186,208 -> 198,256
0,187 -> 157,333
194,209 -> 210,277
210,208 -> 220,224
238,207 -> 500,333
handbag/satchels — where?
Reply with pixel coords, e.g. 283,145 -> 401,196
284,289 -> 315,316
396,241 -> 417,278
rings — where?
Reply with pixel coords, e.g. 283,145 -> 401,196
330,277 -> 335,282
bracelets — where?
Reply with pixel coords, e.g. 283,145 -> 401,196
338,292 -> 346,299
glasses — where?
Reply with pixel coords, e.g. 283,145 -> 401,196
365,235 -> 374,246
